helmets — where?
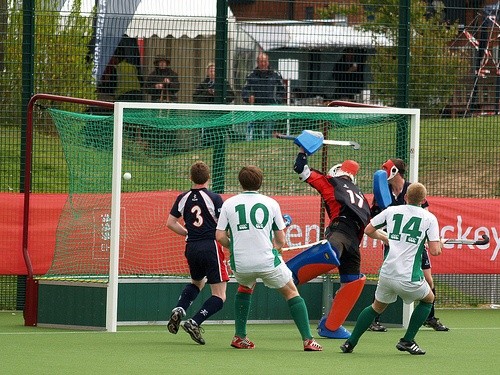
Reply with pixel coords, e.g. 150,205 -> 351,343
328,163 -> 356,184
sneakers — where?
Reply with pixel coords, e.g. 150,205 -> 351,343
167,308 -> 182,334
181,320 -> 205,345
367,321 -> 385,331
396,338 -> 425,355
303,339 -> 323,351
340,340 -> 352,352
231,337 -> 254,349
423,316 -> 448,331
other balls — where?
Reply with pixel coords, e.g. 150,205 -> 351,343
123,172 -> 131,180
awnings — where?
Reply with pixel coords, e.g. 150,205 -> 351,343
236,24 -> 396,56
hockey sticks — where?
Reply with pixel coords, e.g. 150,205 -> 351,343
275,134 -> 361,150
426,235 -> 490,245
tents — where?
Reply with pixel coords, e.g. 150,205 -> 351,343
6,0 -> 238,132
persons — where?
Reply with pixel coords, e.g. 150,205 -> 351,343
166,161 -> 230,345
284,130 -> 393,339
240,53 -> 286,139
144,55 -> 179,151
215,165 -> 324,351
97,59 -> 118,96
339,62 -> 362,99
368,156 -> 450,331
340,183 -> 442,356
192,63 -> 236,148
